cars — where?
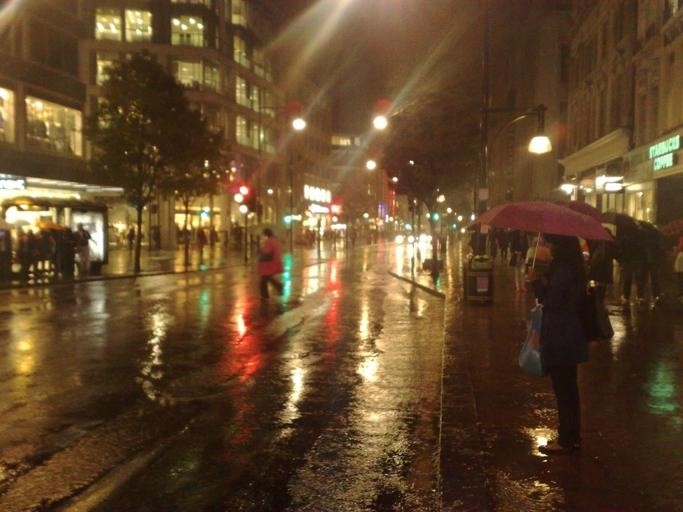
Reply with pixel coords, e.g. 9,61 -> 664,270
394,234 -> 415,248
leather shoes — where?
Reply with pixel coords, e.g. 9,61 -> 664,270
539,441 -> 581,454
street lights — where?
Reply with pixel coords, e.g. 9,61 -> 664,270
255,106 -> 306,252
471,103 -> 552,265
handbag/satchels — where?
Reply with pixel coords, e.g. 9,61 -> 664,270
518,305 -> 543,377
585,292 -> 614,340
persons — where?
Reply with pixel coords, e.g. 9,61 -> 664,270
302,229 -> 339,244
468,225 -> 663,304
524,227 -> 612,455
2,219 -> 91,276
256,227 -> 283,299
126,221 -> 243,249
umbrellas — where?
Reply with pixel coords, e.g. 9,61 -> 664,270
472,198 -> 614,275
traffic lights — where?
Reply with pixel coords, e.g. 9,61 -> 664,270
232,186 -> 251,214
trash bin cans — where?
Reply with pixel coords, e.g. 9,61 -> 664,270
465,255 -> 495,305
